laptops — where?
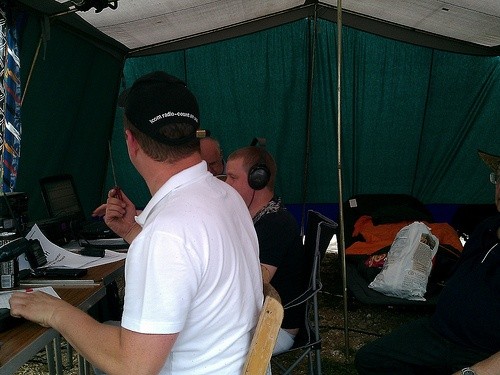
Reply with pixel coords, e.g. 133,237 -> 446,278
37,175 -> 113,237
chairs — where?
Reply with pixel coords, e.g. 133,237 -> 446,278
270,209 -> 339,375
242,264 -> 284,375
336,196 -> 462,306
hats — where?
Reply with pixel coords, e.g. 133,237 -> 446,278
119,71 -> 200,142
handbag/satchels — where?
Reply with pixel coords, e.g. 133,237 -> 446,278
368,222 -> 439,301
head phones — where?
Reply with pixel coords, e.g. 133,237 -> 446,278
248,136 -> 270,190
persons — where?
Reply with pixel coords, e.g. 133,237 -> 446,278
8,70 -> 266,375
90,129 -> 306,375
353,148 -> 500,375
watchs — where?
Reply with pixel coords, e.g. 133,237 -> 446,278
460,366 -> 478,375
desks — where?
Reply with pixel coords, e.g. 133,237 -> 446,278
0,249 -> 125,375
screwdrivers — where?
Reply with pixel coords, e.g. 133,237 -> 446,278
69,248 -> 105,258
108,139 -> 123,201
